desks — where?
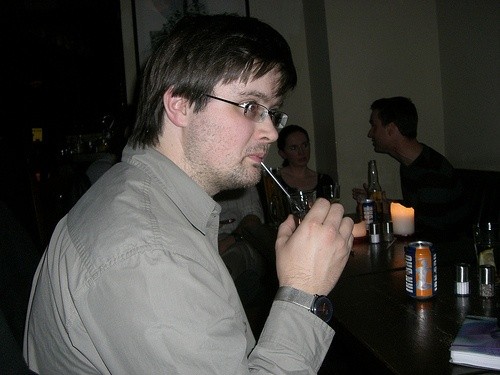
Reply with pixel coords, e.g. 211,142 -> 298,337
327,238 -> 500,375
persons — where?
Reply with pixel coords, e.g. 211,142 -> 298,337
353,95 -> 471,266
22,10 -> 354,375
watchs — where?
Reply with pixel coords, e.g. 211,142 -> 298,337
274,285 -> 335,323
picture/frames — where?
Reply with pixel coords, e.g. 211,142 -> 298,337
132,0 -> 250,79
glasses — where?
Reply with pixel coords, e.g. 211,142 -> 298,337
203,92 -> 288,129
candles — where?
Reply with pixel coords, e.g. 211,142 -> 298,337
390,202 -> 415,235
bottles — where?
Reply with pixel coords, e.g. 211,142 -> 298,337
367,159 -> 384,220
455,262 -> 474,296
473,188 -> 500,289
382,221 -> 393,242
370,222 -> 381,244
478,265 -> 494,298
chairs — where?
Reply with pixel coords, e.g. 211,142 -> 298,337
0,201 -> 39,375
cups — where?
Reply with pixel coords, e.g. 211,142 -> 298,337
323,184 -> 340,204
288,190 -> 316,227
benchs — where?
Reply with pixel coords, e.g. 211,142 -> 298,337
454,169 -> 500,239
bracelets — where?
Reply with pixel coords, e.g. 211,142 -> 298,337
231,230 -> 244,241
